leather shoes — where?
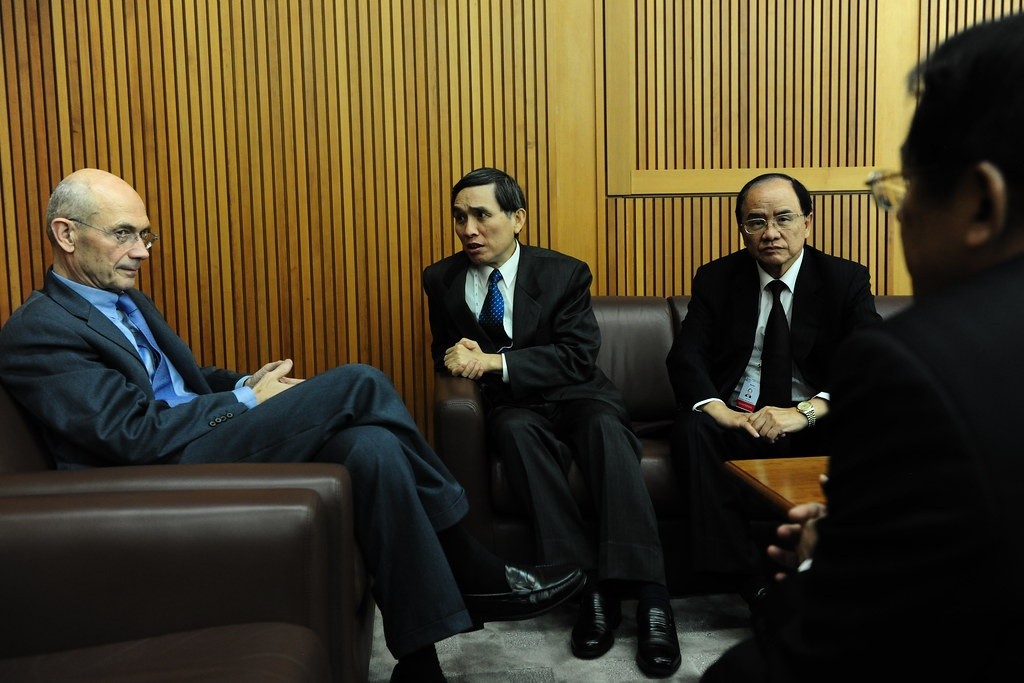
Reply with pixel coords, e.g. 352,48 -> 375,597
461,564 -> 586,633
569,589 -> 622,660
634,598 -> 681,679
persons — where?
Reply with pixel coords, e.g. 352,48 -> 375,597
694,7 -> 1024,682
418,165 -> 682,678
0,167 -> 594,683
665,172 -> 883,609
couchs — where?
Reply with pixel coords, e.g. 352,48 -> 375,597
0,485 -> 335,683
668,293 -> 914,336
0,393 -> 376,683
433,294 -> 678,559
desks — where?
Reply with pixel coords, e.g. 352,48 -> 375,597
723,456 -> 830,510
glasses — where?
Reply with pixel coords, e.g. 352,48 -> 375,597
739,213 -> 806,234
68,217 -> 158,249
864,164 -> 908,210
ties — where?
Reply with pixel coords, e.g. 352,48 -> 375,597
116,292 -> 198,407
478,268 -> 513,354
752,279 -> 791,413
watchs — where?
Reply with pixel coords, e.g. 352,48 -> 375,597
796,401 -> 816,427
